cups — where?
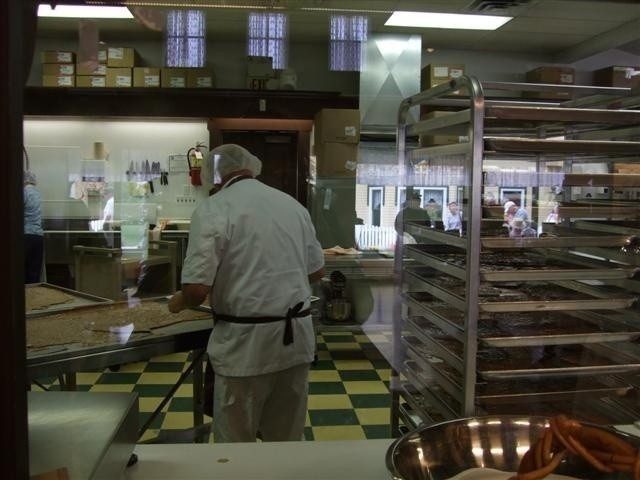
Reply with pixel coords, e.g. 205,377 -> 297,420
94,141 -> 105,160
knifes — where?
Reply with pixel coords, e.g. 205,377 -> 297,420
161,169 -> 168,186
127,160 -> 160,193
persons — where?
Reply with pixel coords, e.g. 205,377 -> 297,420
392,191 -> 568,241
166,142 -> 328,441
23,168 -> 43,285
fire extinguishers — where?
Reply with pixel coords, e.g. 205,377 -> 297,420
186,141 -> 208,187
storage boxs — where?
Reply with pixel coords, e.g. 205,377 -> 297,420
525,66 -> 576,99
608,162 -> 640,174
39,45 -> 304,90
592,65 -> 635,87
313,109 -> 360,143
421,112 -> 470,147
315,143 -> 358,177
421,63 -> 464,97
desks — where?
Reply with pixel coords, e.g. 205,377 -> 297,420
25,283 -> 214,446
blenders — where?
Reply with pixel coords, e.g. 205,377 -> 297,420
322,269 -> 353,321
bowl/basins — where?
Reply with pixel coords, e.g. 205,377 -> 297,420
384,413 -> 640,480
